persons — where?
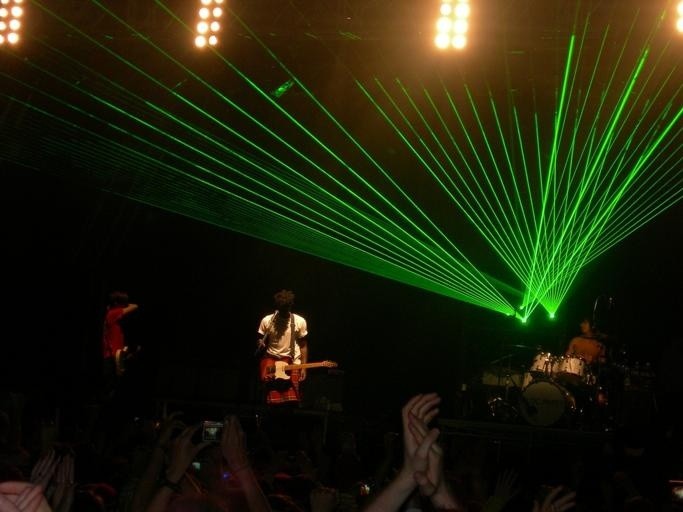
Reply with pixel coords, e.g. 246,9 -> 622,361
255,290 -> 309,402
97,289 -> 138,402
564,316 -> 606,369
2,387 -> 578,510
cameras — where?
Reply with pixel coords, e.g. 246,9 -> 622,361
200,420 -> 226,442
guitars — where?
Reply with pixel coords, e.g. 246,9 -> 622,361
115,344 -> 141,375
260,358 -> 337,383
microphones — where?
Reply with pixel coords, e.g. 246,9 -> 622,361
607,295 -> 613,312
271,310 -> 279,322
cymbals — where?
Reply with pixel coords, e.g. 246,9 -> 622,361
512,343 -> 531,353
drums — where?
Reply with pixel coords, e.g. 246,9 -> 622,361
557,356 -> 587,385
521,381 -> 576,425
530,351 -> 554,380
520,372 -> 534,390
